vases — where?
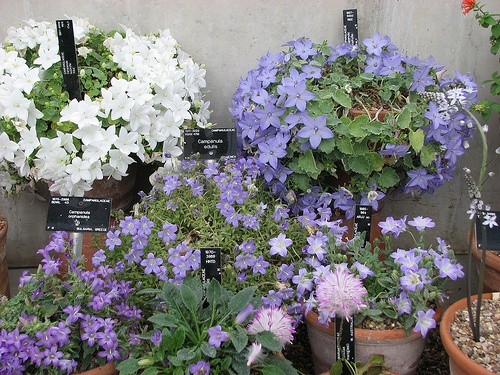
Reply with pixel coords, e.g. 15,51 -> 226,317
298,299 -> 442,375
0,214 -> 10,301
47,153 -> 138,213
56,215 -> 120,280
69,348 -> 129,375
466,227 -> 500,293
439,292 -> 500,375
330,213 -> 386,265
331,160 -> 394,214
350,106 -> 394,153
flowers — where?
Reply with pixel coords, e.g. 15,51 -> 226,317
0,0 -> 500,375
